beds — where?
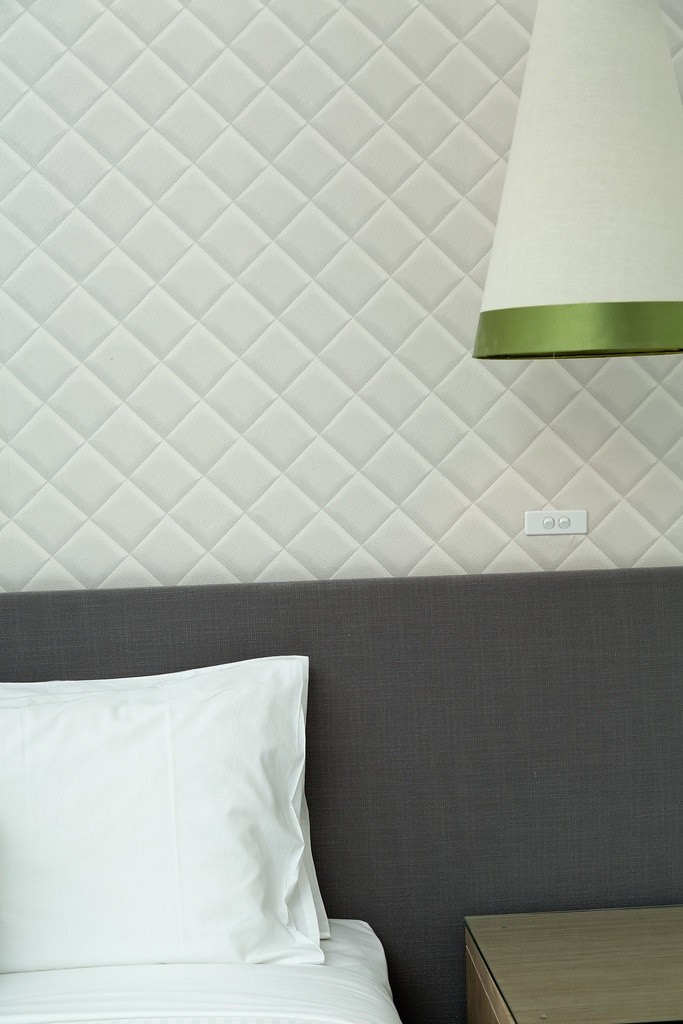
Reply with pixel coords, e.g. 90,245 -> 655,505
0,920 -> 399,1024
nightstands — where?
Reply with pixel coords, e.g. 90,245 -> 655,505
464,904 -> 683,1023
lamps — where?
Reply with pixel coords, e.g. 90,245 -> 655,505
472,1 -> 683,357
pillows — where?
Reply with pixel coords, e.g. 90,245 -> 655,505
0,656 -> 330,968
